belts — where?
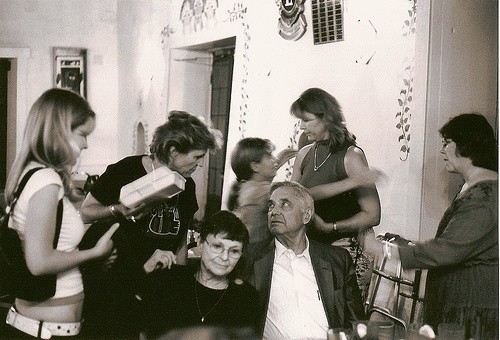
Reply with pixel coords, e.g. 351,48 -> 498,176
6,306 -> 81,339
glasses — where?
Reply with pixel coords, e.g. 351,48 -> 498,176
203,237 -> 243,259
441,140 -> 456,149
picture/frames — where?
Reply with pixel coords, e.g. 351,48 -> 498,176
51,47 -> 88,101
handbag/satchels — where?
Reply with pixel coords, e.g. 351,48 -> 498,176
0,167 -> 63,303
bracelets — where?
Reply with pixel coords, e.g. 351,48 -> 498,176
108,204 -> 119,219
333,221 -> 337,231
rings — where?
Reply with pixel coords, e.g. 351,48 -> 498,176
131,215 -> 136,223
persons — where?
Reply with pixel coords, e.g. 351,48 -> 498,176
378,112 -> 500,340
77,87 -> 382,340
0,87 -> 120,340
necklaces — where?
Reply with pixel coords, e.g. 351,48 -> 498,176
151,159 -> 155,171
194,270 -> 229,323
313,142 -> 332,171
161,255 -> 165,259
